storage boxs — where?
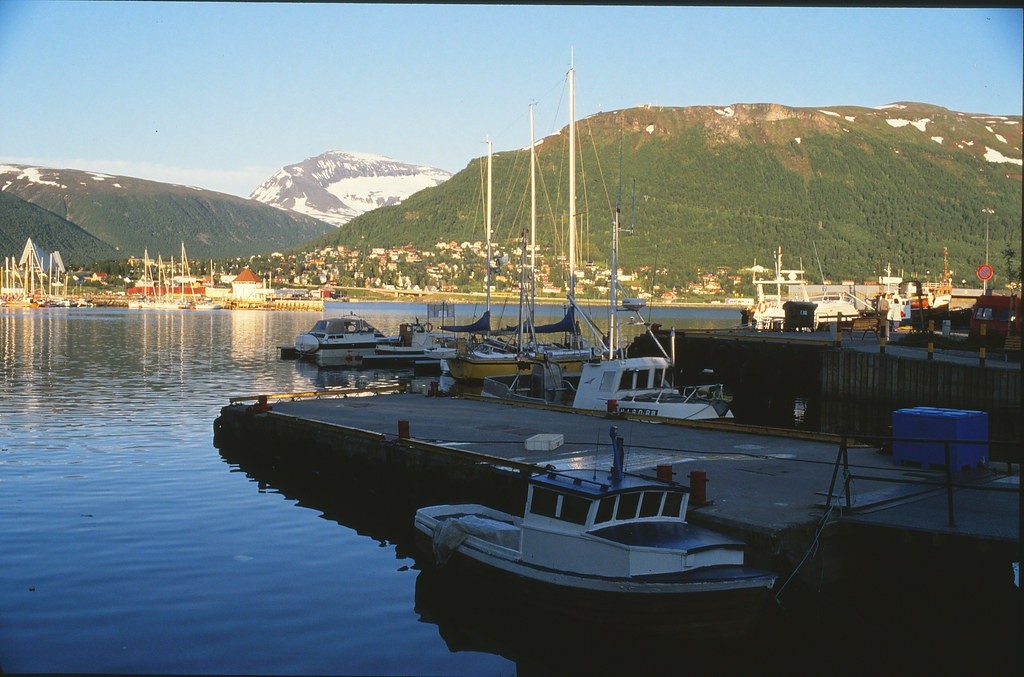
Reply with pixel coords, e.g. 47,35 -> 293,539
411,380 -> 431,392
526,434 -> 564,451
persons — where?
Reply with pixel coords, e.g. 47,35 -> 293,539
878,294 -> 890,312
887,299 -> 902,331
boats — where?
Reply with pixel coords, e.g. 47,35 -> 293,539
414,422 -> 781,627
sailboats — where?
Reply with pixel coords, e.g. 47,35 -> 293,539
277,38 -> 736,426
0,241 -> 224,310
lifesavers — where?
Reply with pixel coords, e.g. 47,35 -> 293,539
423,322 -> 433,333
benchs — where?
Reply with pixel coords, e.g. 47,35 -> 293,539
841,318 -> 883,343
1004,336 -> 1021,362
